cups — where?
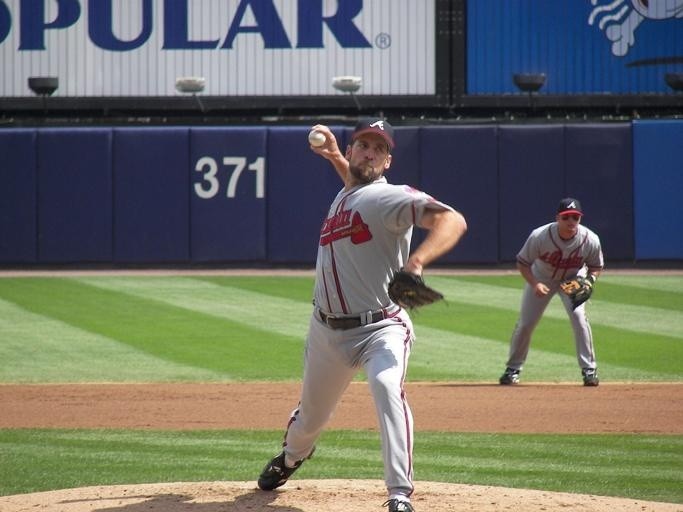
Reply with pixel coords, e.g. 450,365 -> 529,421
560,214 -> 580,222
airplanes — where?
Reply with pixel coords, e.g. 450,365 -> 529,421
388,267 -> 448,310
561,275 -> 593,311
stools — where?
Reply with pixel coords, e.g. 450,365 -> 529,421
312,300 -> 383,330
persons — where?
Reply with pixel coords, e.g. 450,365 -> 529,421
258,119 -> 467,511
497,197 -> 605,385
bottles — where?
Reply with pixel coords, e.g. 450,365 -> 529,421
554,198 -> 585,217
352,117 -> 397,152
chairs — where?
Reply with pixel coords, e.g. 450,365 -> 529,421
499,367 -> 520,385
382,499 -> 416,512
258,445 -> 316,492
581,368 -> 600,387
